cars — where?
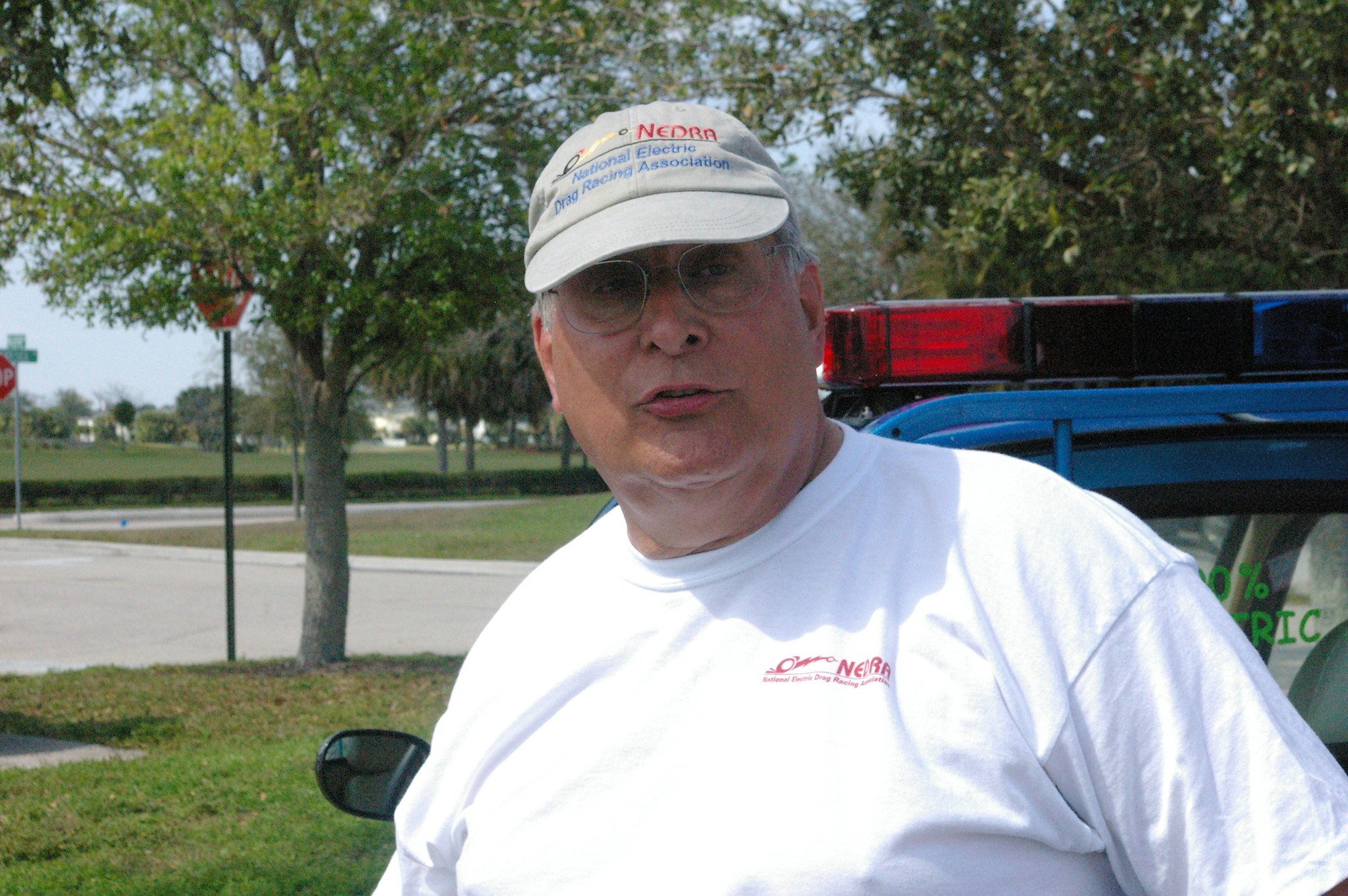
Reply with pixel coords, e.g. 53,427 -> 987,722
822,287 -> 1346,769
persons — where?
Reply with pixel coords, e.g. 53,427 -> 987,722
371,102 -> 1348,895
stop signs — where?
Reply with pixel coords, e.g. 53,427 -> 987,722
0,353 -> 16,398
193,226 -> 254,332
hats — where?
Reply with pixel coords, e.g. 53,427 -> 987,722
523,101 -> 799,294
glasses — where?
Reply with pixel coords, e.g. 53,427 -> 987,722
546,240 -> 796,335
